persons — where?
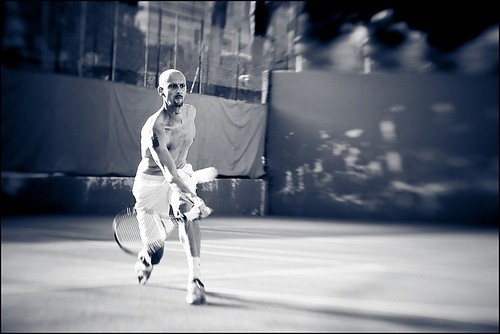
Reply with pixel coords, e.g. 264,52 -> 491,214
132,68 -> 211,304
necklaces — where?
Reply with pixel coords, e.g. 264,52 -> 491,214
164,107 -> 182,114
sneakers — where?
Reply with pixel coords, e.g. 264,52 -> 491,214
186,278 -> 206,305
137,251 -> 153,285
